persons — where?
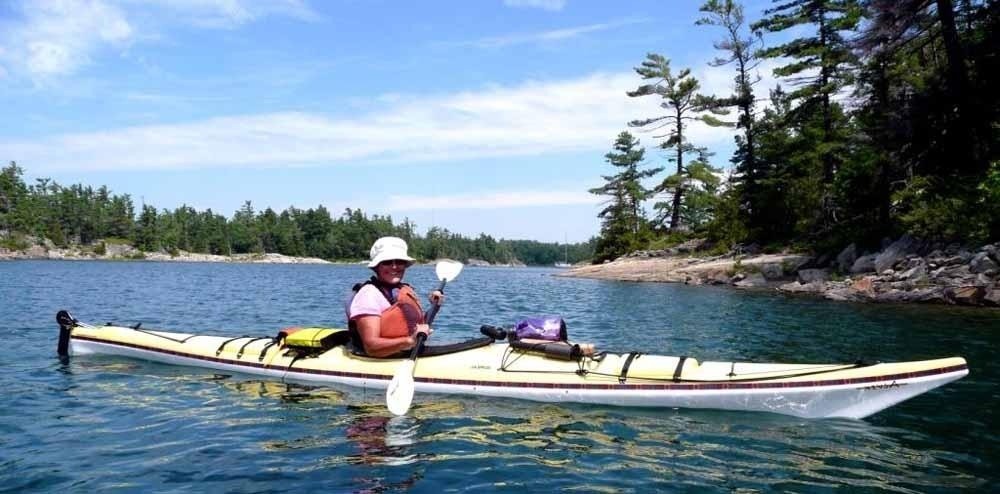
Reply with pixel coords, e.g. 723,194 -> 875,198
348,235 -> 446,358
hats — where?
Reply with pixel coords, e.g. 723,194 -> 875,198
365,236 -> 418,272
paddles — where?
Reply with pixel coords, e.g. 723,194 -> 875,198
386,261 -> 464,417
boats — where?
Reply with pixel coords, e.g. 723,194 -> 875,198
49,294 -> 975,428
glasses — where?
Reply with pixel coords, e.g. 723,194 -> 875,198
379,259 -> 407,266
